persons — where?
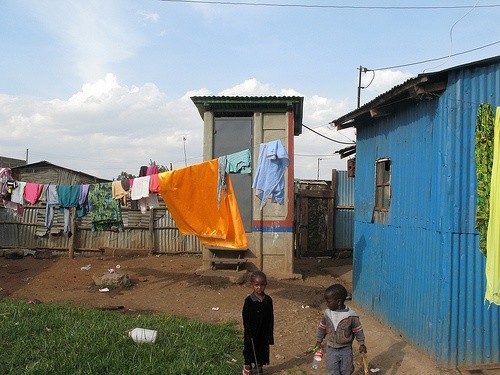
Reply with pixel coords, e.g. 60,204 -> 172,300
311,284 -> 368,375
241,271 -> 275,375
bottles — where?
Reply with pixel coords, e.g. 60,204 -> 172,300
310,344 -> 324,372
128,328 -> 157,344
